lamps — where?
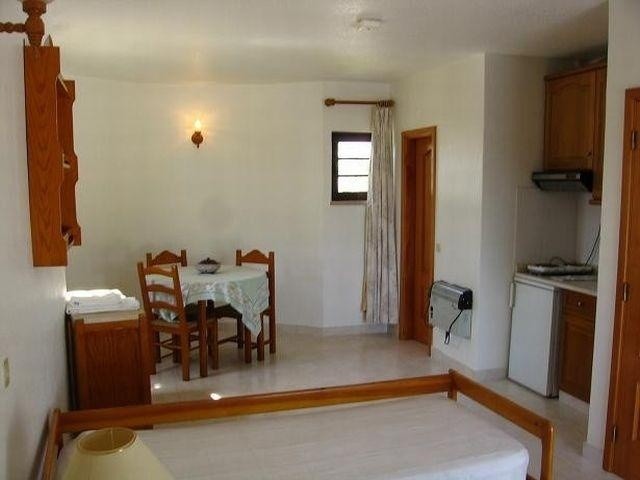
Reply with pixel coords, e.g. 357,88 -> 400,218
352,17 -> 382,35
192,121 -> 205,149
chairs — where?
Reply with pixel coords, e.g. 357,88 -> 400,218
135,247 -> 276,381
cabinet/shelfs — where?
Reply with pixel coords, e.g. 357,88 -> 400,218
24,45 -> 82,267
556,286 -> 597,404
543,59 -> 607,172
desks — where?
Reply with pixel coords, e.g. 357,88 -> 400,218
68,308 -> 153,409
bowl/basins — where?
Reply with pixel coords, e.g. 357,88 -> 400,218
194,263 -> 221,274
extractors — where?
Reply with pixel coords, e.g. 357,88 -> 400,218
530,172 -> 594,193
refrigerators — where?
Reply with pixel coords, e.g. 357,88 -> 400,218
507,277 -> 559,399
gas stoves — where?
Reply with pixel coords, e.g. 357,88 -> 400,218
525,263 -> 592,276
